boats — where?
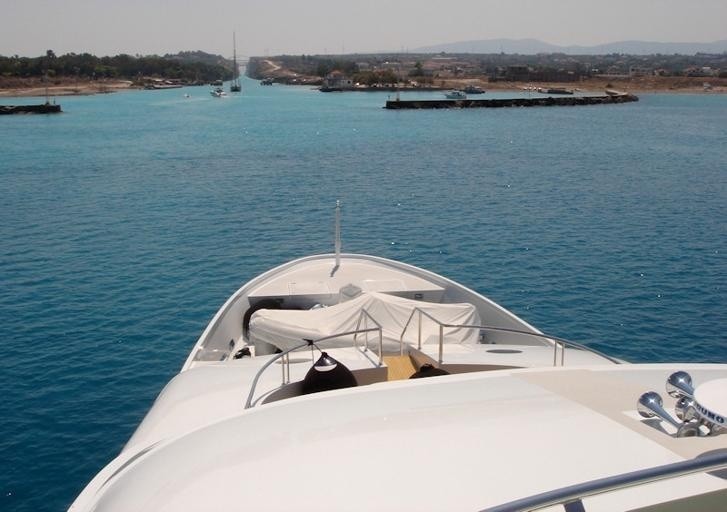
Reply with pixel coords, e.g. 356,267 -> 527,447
66,198 -> 727,510
210,78 -> 224,96
320,86 -> 341,92
548,86 -> 573,93
259,78 -> 274,86
445,90 -> 467,100
464,85 -> 485,94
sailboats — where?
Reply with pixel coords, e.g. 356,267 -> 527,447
232,30 -> 240,92
